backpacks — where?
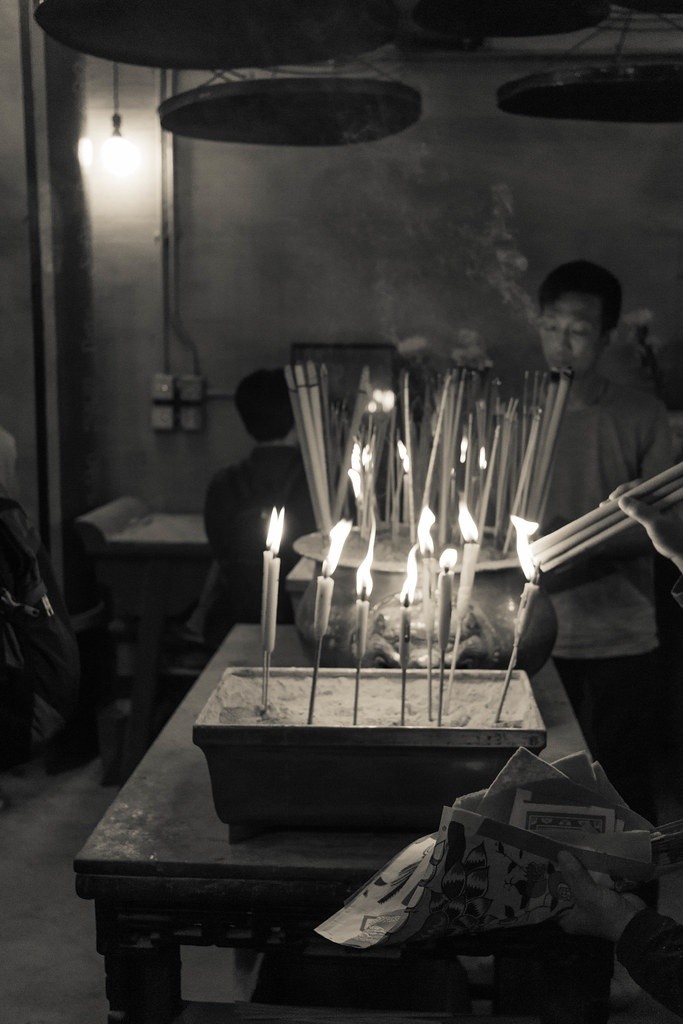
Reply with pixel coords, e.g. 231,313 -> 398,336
224,455 -> 310,624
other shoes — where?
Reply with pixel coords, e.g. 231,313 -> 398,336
459,953 -> 495,972
608,961 -> 641,1008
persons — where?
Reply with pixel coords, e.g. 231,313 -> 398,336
556,850 -> 683,1019
512,258 -> 683,913
0,429 -> 82,811
200,368 -> 318,655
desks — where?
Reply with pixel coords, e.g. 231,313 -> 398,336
75,623 -> 595,1024
75,492 -> 212,790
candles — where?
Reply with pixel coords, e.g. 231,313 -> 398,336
257,504 -> 288,710
397,496 -> 481,727
351,531 -> 374,729
494,516 -> 543,724
306,518 -> 353,726
